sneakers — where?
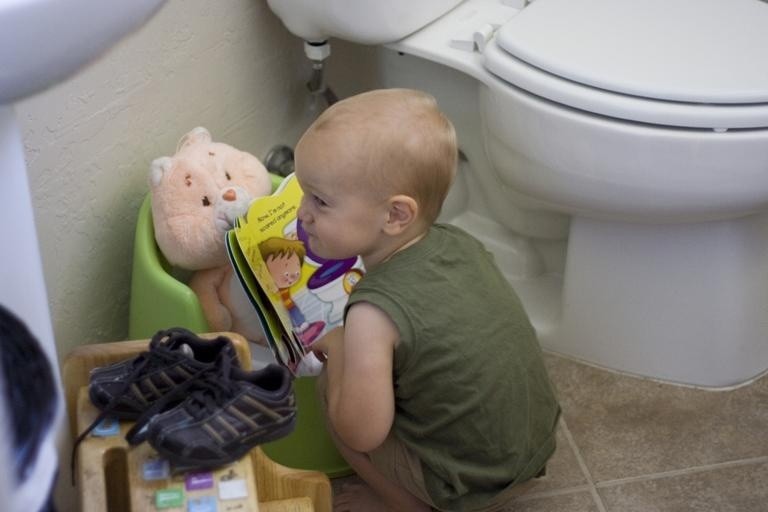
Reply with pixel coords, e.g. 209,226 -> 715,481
88,327 -> 240,421
148,363 -> 296,471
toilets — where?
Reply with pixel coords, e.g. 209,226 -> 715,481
266,0 -> 767,391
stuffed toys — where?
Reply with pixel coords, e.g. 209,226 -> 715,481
149,125 -> 272,345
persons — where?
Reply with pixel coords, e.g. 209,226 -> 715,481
293,87 -> 562,511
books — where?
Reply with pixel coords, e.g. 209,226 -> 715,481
225,171 -> 367,378
